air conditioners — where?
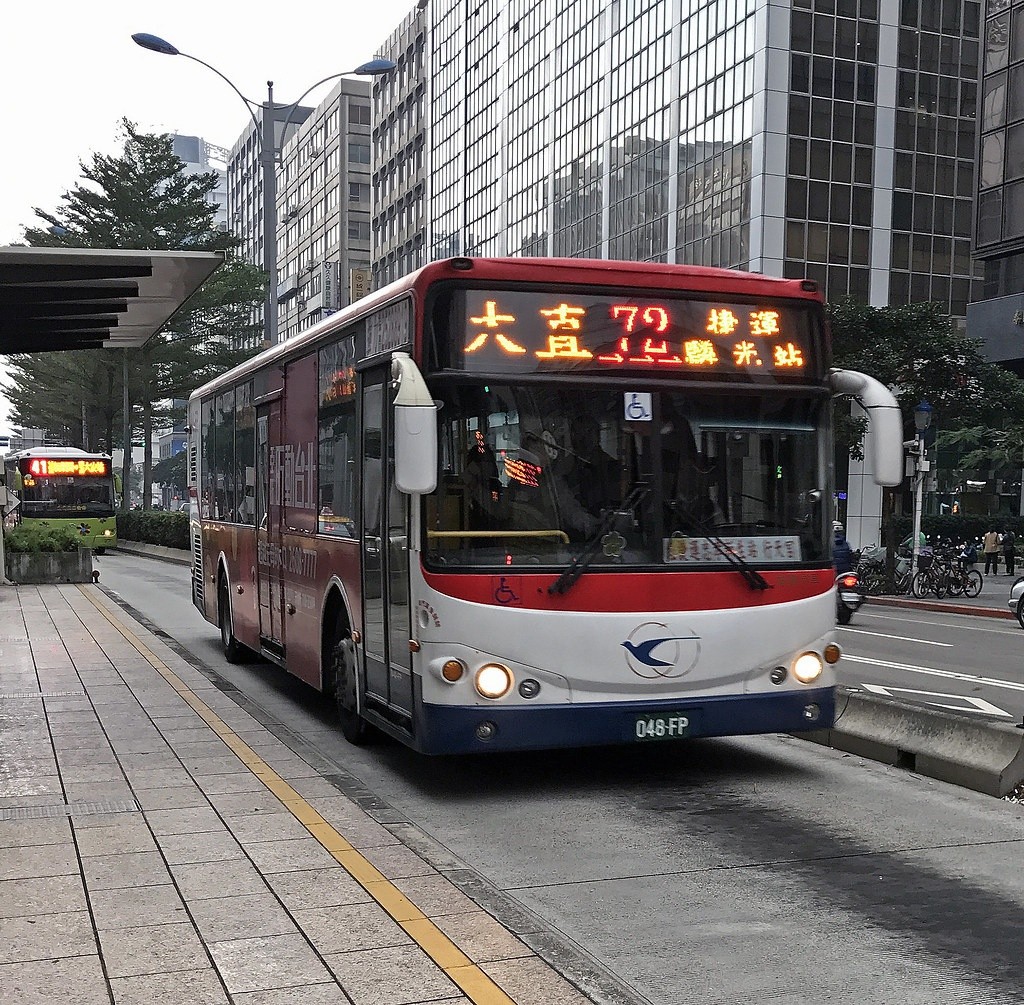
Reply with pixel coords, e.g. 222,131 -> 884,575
281,214 -> 286,222
288,206 -> 296,216
306,260 -> 311,268
309,146 -> 314,156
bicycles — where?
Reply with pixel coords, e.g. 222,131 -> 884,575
851,533 -> 987,599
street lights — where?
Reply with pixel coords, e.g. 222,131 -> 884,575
131,31 -> 401,349
912,399 -> 933,597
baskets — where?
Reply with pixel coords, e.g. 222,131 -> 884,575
917,555 -> 932,567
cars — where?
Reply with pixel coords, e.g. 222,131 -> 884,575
178,503 -> 190,514
1007,573 -> 1024,628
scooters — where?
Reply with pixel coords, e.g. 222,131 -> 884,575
835,571 -> 865,626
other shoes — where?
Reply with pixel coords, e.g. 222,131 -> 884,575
984,574 -> 987,576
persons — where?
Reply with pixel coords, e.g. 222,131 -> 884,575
983,525 -> 1000,576
557,417 -> 623,530
832,520 -> 853,577
457,443 -> 508,531
957,539 -> 977,571
1001,526 -> 1015,576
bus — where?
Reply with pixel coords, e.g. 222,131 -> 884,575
4,447 -> 123,554
184,255 -> 904,756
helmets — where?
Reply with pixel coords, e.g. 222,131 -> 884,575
832,521 -> 843,531
